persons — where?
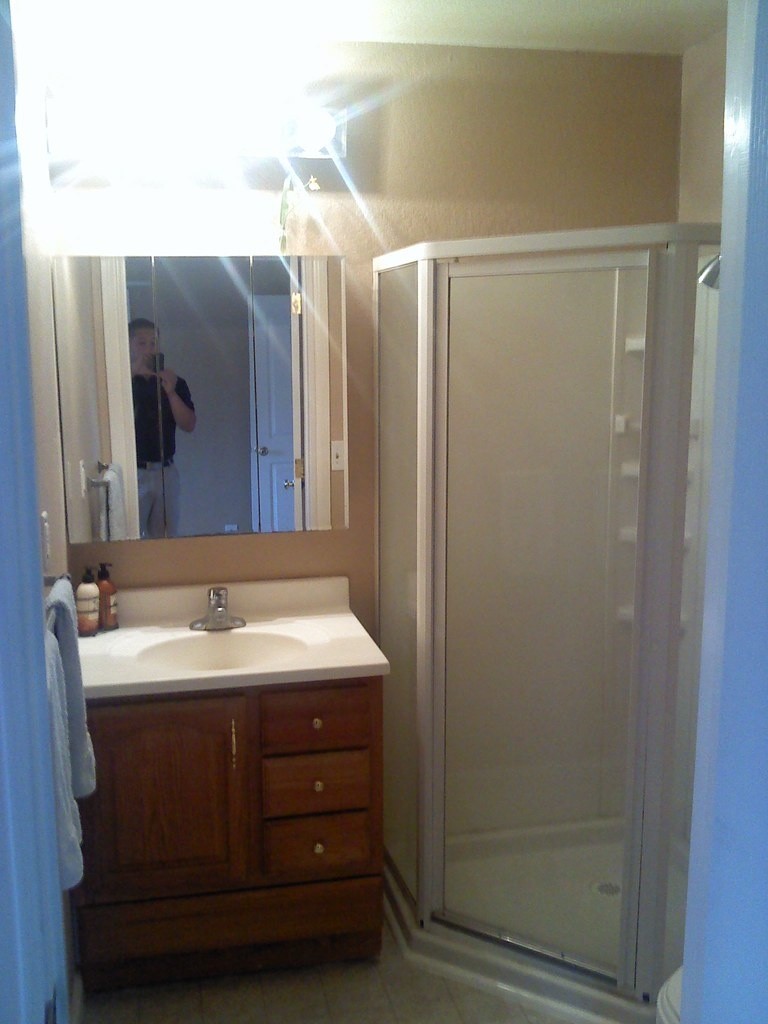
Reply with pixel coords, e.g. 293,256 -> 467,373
129,318 -> 197,538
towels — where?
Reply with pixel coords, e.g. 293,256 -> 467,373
42,629 -> 85,891
93,463 -> 129,541
44,581 -> 96,799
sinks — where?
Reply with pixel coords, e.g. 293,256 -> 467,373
137,628 -> 309,671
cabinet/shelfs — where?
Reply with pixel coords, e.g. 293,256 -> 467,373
68,674 -> 385,998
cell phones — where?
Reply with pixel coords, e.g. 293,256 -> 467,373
152,352 -> 165,376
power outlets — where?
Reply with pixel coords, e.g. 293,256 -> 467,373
224,524 -> 237,535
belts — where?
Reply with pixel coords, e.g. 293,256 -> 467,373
137,459 -> 174,469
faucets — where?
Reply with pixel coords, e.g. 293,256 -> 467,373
189,587 -> 246,631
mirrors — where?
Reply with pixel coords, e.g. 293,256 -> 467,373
52,252 -> 344,544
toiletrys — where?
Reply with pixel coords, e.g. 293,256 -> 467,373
74,569 -> 101,635
97,563 -> 119,630
